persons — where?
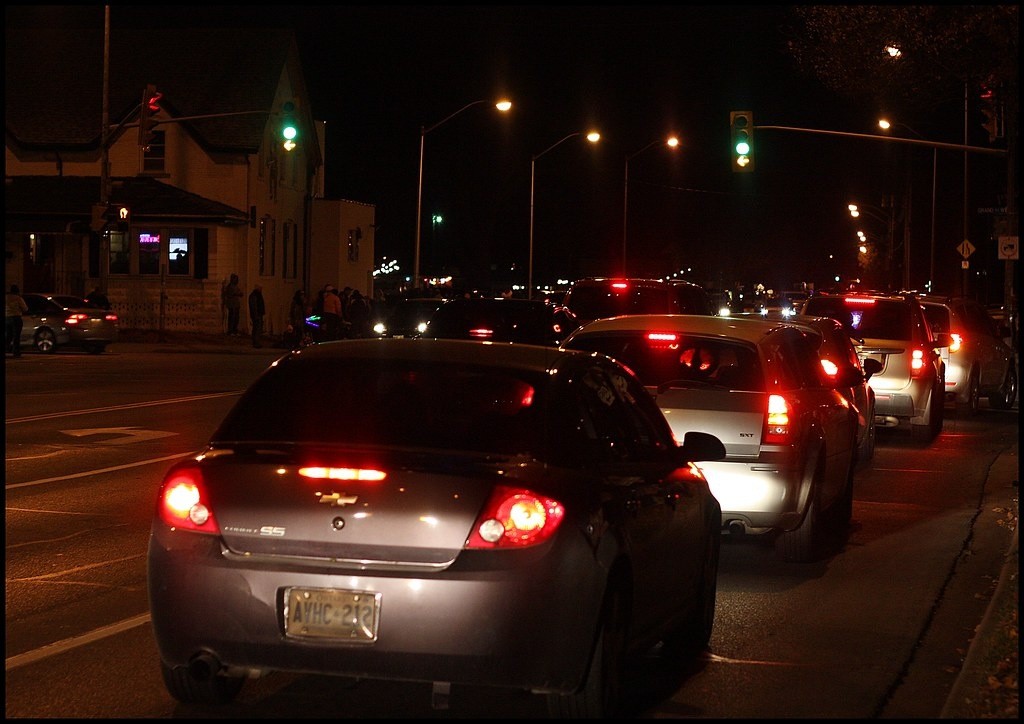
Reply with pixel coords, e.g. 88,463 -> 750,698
86,285 -> 110,351
249,284 -> 266,348
225,274 -> 244,337
5,284 -> 29,358
287,284 -> 387,348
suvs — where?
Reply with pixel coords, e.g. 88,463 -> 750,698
802,293 -> 955,444
565,276 -> 717,329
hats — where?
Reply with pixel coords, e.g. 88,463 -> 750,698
326,286 -> 334,291
345,287 -> 353,291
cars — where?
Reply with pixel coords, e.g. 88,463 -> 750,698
719,291 -> 807,319
561,315 -> 883,564
18,293 -> 119,354
986,302 -> 1005,320
913,300 -> 982,414
910,296 -> 1017,414
370,298 -> 579,348
147,339 -> 727,724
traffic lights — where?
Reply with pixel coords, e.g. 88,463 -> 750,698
430,212 -> 443,242
981,77 -> 1004,143
140,84 -> 166,147
729,111 -> 755,173
278,101 -> 300,154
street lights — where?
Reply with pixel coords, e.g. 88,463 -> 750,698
414,97 -> 512,283
622,136 -> 679,278
529,130 -> 601,298
878,119 -> 937,295
849,203 -> 896,294
